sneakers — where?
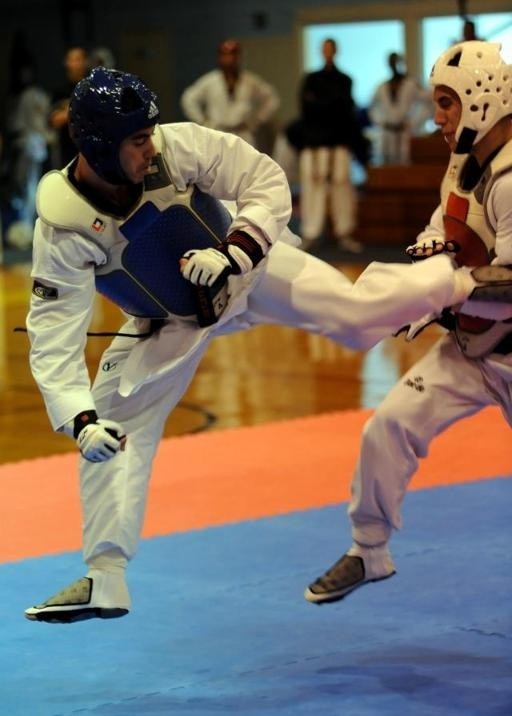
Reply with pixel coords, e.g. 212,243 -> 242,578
456,266 -> 511,302
25,570 -> 128,623
306,544 -> 395,604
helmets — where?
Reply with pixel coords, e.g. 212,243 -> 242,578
68,66 -> 161,167
429,41 -> 512,146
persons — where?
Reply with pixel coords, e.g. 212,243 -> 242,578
368,52 -> 434,163
284,38 -> 367,253
12,47 -> 112,221
181,35 -> 281,149
301,36 -> 512,606
23,63 -> 511,621
450,20 -> 487,45
